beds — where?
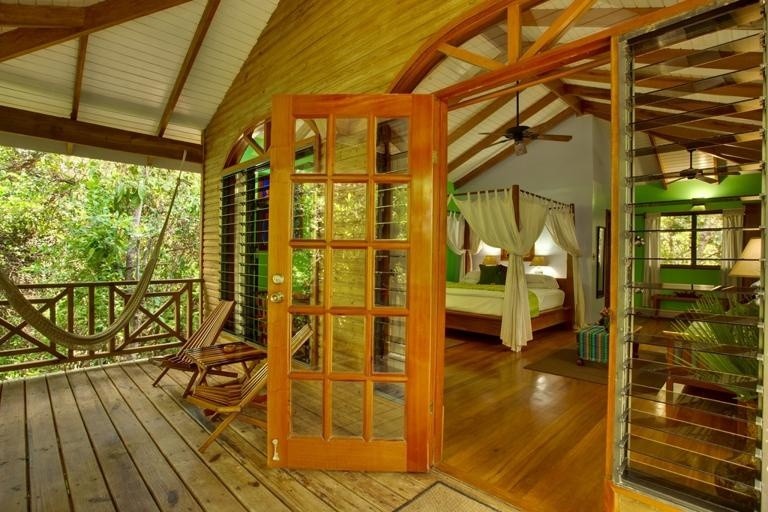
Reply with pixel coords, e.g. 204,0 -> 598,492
446,277 -> 583,350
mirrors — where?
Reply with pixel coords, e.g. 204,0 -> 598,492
596,226 -> 606,297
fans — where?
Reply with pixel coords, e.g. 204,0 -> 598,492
479,77 -> 573,158
631,146 -> 765,186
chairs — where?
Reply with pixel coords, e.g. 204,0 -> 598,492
148,299 -> 315,453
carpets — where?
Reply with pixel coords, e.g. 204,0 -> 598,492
524,338 -> 670,397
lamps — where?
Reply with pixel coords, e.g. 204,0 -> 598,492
729,237 -> 763,279
529,255 -> 550,275
481,256 -> 498,269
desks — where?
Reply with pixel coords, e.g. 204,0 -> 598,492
575,321 -> 644,368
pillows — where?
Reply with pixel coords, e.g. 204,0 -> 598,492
478,263 -> 507,285
